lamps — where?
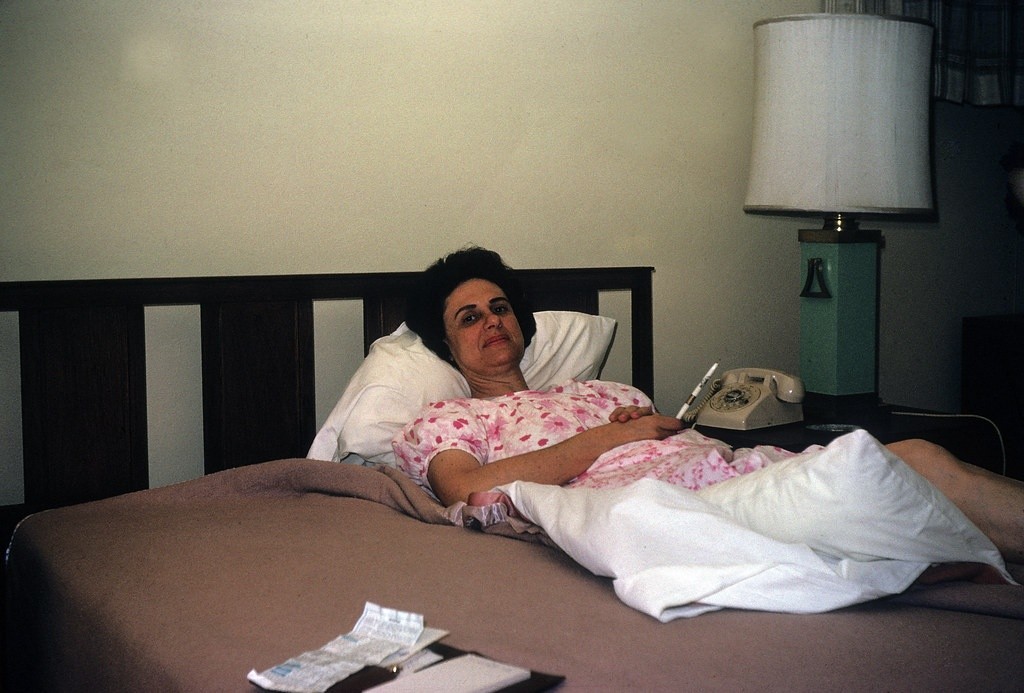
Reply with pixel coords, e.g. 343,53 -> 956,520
740,12 -> 939,425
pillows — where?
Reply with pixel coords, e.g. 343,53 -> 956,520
342,311 -> 618,468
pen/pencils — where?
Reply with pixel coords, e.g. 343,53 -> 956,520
676,358 -> 723,419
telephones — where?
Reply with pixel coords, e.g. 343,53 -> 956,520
697,367 -> 805,431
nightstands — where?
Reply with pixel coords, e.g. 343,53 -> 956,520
686,404 -> 996,467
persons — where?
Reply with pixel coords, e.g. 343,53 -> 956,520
391,246 -> 1024,554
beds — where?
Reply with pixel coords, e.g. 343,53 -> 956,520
0,265 -> 1024,693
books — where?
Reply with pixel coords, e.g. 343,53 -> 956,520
245,602 -> 566,693
